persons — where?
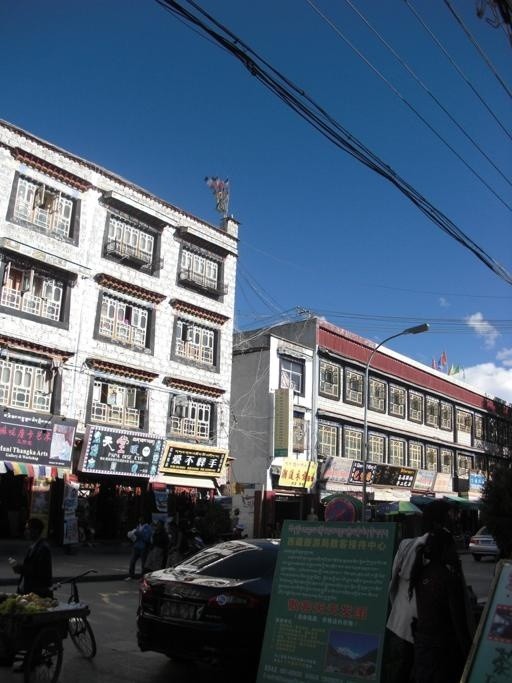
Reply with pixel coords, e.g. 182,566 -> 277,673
405,527 -> 474,682
11,515 -> 54,673
123,513 -> 249,581
385,501 -> 462,682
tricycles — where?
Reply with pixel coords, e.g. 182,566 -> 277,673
0,568 -> 100,683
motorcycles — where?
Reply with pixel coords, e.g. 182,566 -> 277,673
178,527 -> 205,557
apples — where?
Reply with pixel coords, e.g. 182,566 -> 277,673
0,590 -> 59,613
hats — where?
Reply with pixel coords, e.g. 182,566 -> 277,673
127,529 -> 137,543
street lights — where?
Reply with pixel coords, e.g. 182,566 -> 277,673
360,323 -> 431,522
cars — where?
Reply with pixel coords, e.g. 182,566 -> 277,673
468,526 -> 501,561
135,537 -> 281,668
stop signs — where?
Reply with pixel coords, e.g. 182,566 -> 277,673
325,497 -> 356,523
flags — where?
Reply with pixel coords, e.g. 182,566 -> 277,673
432,351 -> 464,378
